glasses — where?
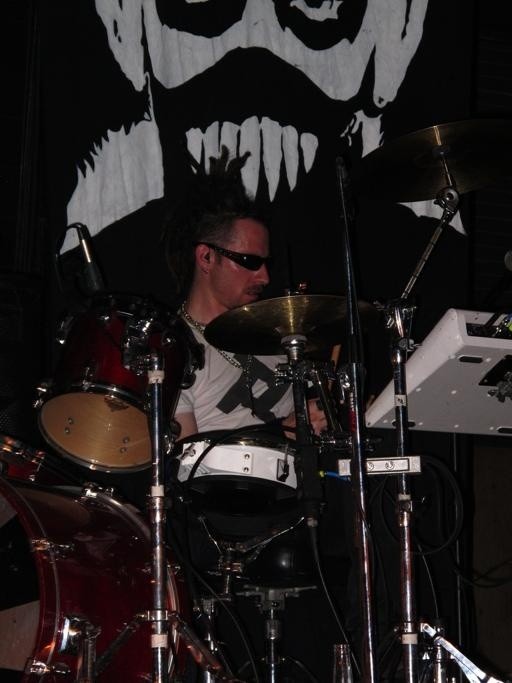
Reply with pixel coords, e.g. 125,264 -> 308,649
194,242 -> 273,270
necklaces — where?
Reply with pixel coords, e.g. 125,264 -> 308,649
180,301 -> 254,388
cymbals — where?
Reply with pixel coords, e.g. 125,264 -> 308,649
203,295 -> 376,356
342,117 -> 511,211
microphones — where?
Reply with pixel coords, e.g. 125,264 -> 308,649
335,156 -> 352,193
76,224 -> 109,311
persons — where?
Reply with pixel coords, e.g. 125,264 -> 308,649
155,210 -> 344,449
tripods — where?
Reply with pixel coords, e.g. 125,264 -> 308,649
346,332 -> 508,682
91,361 -> 232,682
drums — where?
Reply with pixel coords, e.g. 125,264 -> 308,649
174,435 -> 301,534
37,293 -> 204,473
1,433 -> 66,485
0,474 -> 186,681
